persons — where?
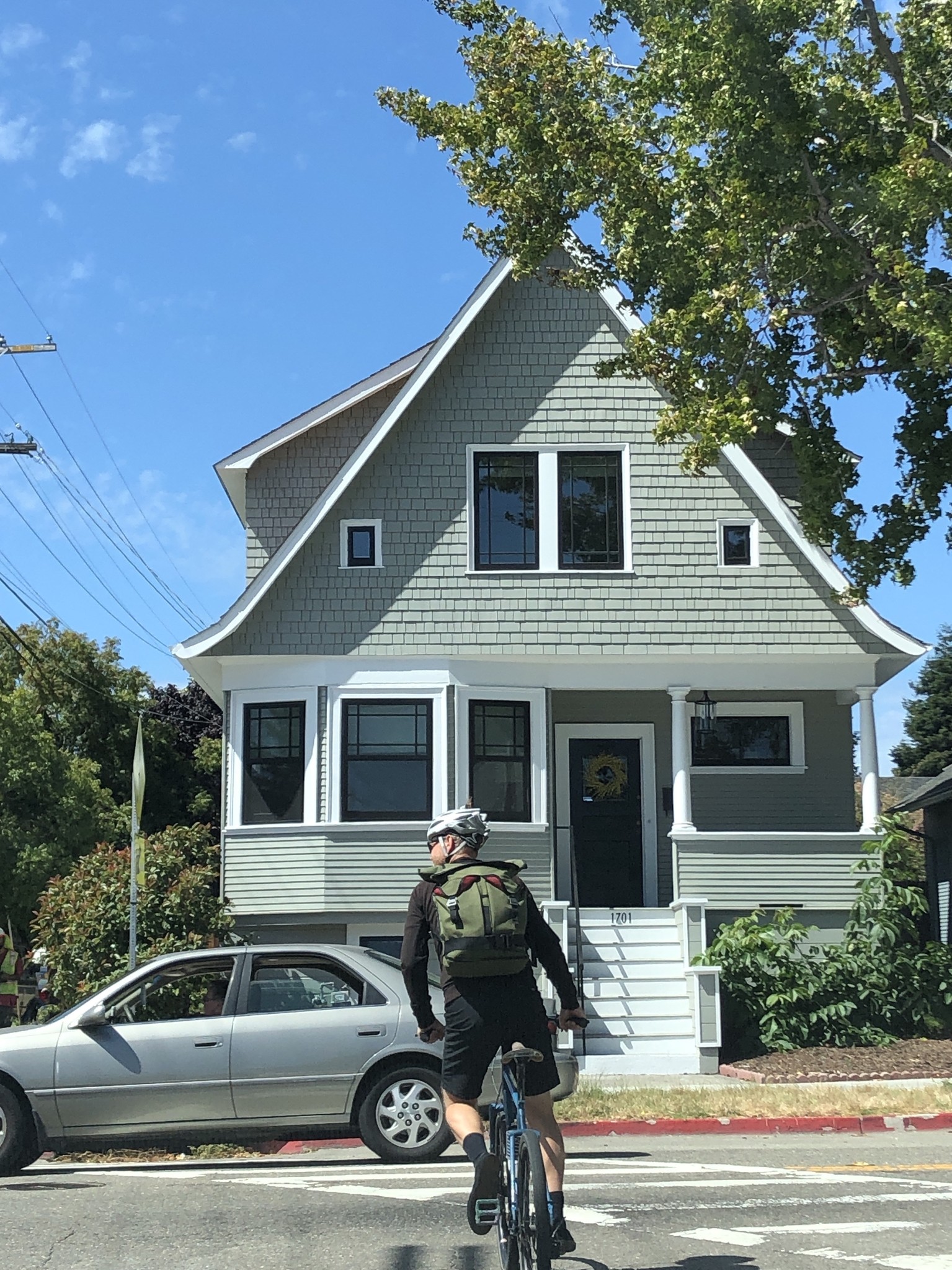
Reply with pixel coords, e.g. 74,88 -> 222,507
204,979 -> 229,1016
0,927 -> 60,1029
400,807 -> 587,1252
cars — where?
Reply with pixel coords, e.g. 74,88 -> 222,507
0,941 -> 578,1179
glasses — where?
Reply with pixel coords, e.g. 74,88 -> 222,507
427,838 -> 457,854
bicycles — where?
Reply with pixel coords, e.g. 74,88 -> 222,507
417,1014 -> 592,1270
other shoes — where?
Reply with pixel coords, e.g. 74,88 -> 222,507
467,1153 -> 502,1235
550,1227 -> 576,1255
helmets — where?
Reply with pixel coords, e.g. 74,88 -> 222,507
427,808 -> 491,851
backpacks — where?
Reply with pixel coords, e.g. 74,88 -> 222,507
417,859 -> 529,977
